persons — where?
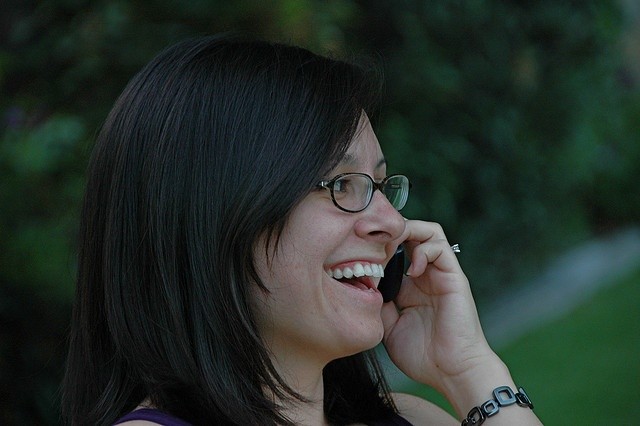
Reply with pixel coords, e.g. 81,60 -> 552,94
66,31 -> 547,426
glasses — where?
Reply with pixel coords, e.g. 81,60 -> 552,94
313,171 -> 413,214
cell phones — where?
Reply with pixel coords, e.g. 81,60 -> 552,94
378,246 -> 406,303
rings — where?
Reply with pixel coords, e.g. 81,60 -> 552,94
451,243 -> 461,254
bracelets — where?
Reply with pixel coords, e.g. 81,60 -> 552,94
461,386 -> 534,425
